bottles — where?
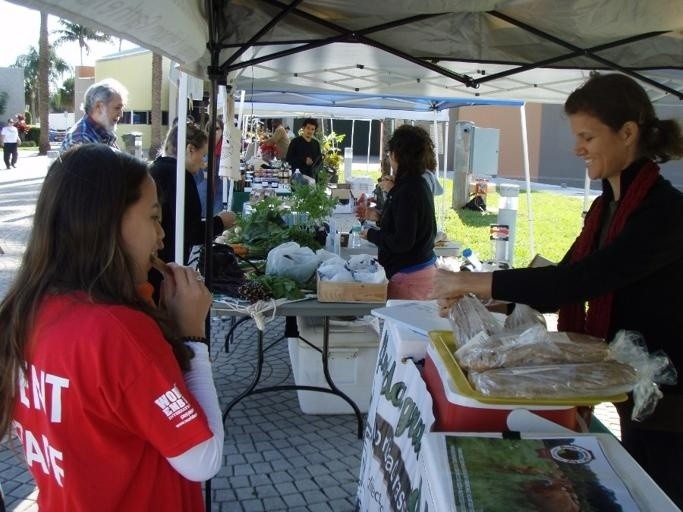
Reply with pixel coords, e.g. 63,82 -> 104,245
292,168 -> 302,184
260,177 -> 279,182
271,179 -> 279,188
459,248 -> 478,273
262,179 -> 269,188
254,177 -> 261,182
255,168 -> 292,177
351,217 -> 362,249
278,178 -> 291,191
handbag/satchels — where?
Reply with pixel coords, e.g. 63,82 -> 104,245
198,243 -> 246,283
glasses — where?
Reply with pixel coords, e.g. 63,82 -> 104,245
215,126 -> 220,130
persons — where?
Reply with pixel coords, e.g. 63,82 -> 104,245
287,119 -> 323,183
171,115 -> 197,125
205,119 -> 224,158
1,117 -> 21,169
147,123 -> 238,302
432,73 -> 682,511
0,143 -> 224,512
357,125 -> 437,302
285,127 -> 295,141
378,137 -> 445,200
261,119 -> 288,161
58,79 -> 126,157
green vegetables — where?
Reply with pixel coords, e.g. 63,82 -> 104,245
251,193 -> 290,218
293,171 -> 338,220
233,227 -> 320,258
238,273 -> 305,304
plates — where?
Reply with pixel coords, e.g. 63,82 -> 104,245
429,324 -> 629,405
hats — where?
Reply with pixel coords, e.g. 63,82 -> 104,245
8,118 -> 13,122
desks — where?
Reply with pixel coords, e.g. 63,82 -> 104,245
210,196 -> 386,440
384,320 -> 680,512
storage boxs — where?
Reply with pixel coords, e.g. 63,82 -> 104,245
422,343 -> 575,432
288,314 -> 380,414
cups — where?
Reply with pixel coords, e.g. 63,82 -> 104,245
340,234 -> 354,250
